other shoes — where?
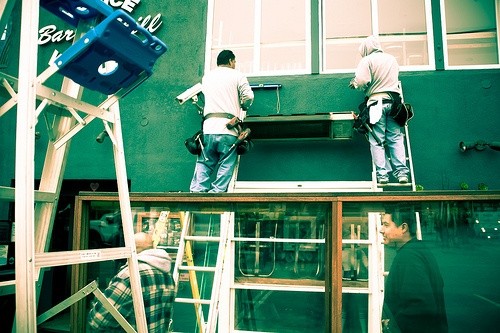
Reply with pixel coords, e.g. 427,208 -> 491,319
380,179 -> 388,184
399,177 -> 408,182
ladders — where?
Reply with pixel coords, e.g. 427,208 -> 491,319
172,110 -> 246,333
136,210 -> 205,333
367,81 -> 422,333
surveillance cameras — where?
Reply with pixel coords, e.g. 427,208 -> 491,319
175,82 -> 204,105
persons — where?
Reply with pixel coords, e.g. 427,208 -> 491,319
380,210 -> 448,333
88,231 -> 175,333
190,50 -> 254,192
350,35 -> 410,184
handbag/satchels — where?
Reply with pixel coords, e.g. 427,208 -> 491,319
389,100 -> 413,126
